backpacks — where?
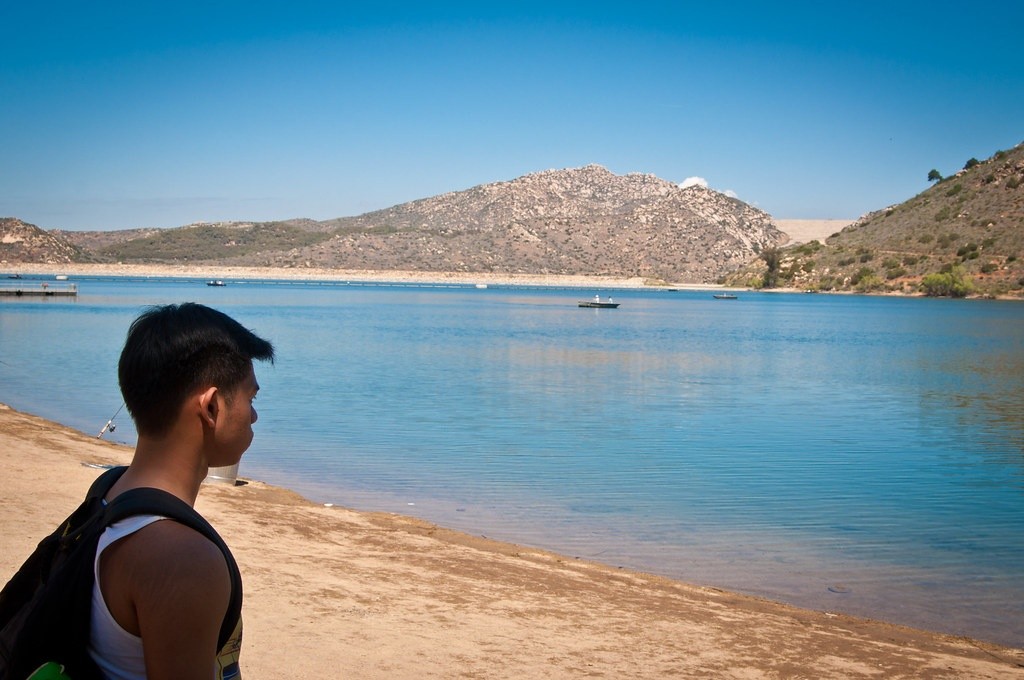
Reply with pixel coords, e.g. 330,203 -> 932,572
0,466 -> 242,680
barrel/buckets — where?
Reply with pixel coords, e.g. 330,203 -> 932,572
202,462 -> 239,486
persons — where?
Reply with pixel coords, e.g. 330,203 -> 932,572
608,296 -> 612,303
87,302 -> 274,680
594,295 -> 599,303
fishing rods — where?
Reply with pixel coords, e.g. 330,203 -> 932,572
96,403 -> 125,439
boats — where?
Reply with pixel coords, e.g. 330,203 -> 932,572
668,289 -> 680,291
9,274 -> 21,280
578,301 -> 621,309
713,294 -> 737,300
805,289 -> 815,293
206,281 -> 226,286
